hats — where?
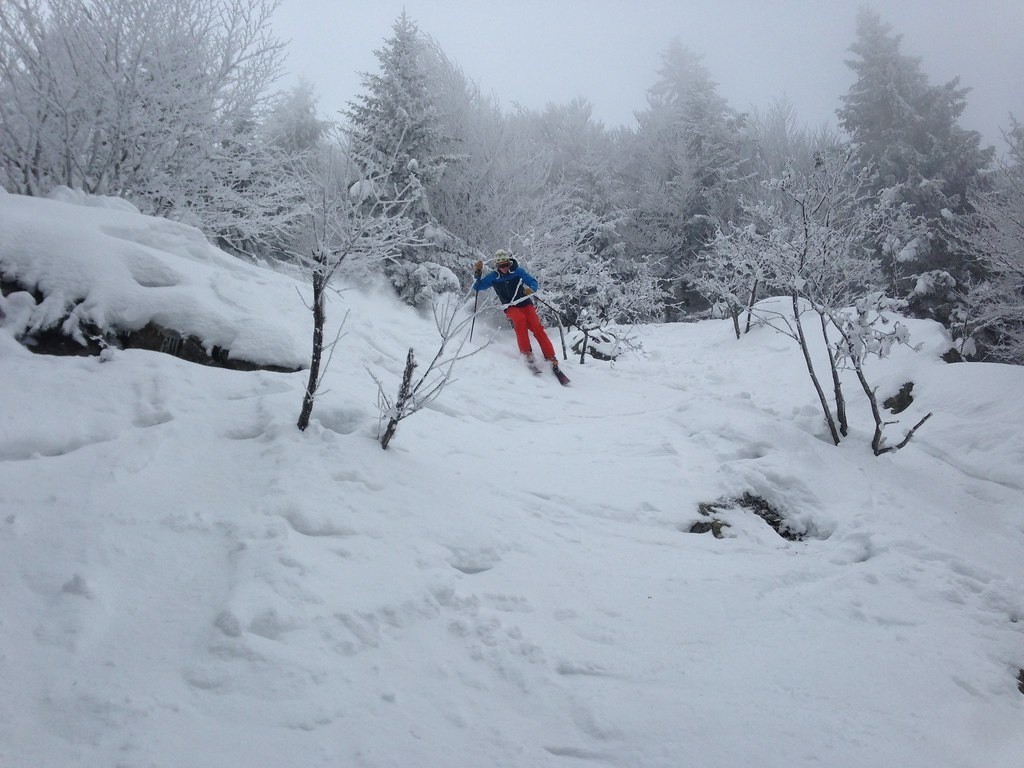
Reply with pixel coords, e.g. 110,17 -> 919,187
494,249 -> 509,263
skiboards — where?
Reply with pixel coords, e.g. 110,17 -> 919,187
523,356 -> 571,389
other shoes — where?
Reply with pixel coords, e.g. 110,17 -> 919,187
522,350 -> 534,362
547,357 -> 559,366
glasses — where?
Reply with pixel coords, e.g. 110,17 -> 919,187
497,262 -> 510,268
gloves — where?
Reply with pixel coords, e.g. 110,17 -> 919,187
523,289 -> 531,295
475,262 -> 483,270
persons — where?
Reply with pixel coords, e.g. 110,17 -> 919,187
472,249 -> 559,368
